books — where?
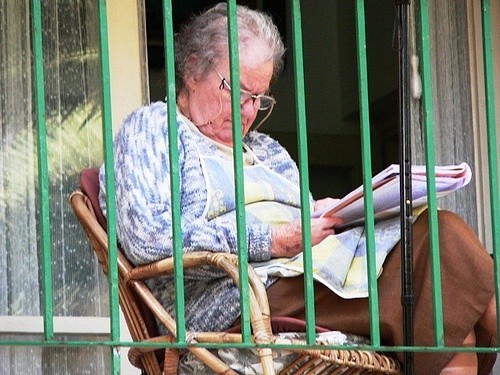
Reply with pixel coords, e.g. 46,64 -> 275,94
315,162 -> 473,228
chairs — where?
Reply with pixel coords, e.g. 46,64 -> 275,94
70,169 -> 402,375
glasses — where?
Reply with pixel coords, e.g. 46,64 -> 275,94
210,60 -> 278,112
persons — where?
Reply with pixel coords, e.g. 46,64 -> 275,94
98,3 -> 500,375
